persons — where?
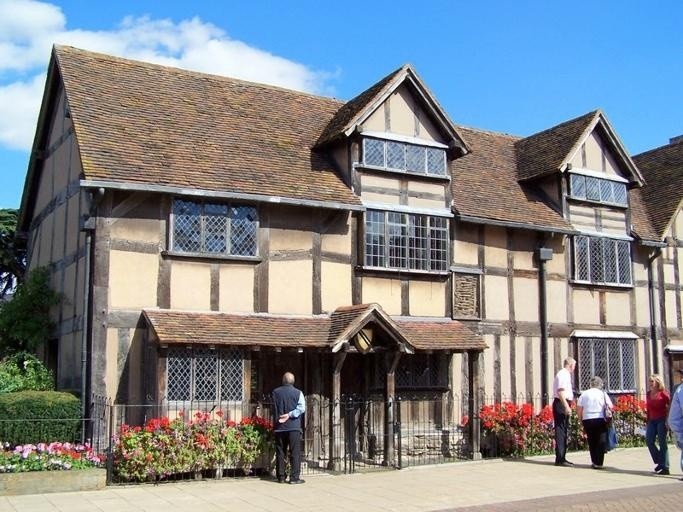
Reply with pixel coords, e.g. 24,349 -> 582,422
576,376 -> 613,469
552,357 -> 576,467
267,372 -> 308,486
644,369 -> 683,482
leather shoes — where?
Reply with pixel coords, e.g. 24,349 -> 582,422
555,459 -> 575,466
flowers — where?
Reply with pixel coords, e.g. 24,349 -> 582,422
461,391 -> 648,458
0,438 -> 106,474
106,408 -> 275,485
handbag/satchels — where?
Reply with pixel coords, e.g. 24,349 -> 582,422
603,390 -> 613,422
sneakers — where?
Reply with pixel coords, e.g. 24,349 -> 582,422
655,464 -> 671,476
278,479 -> 306,484
